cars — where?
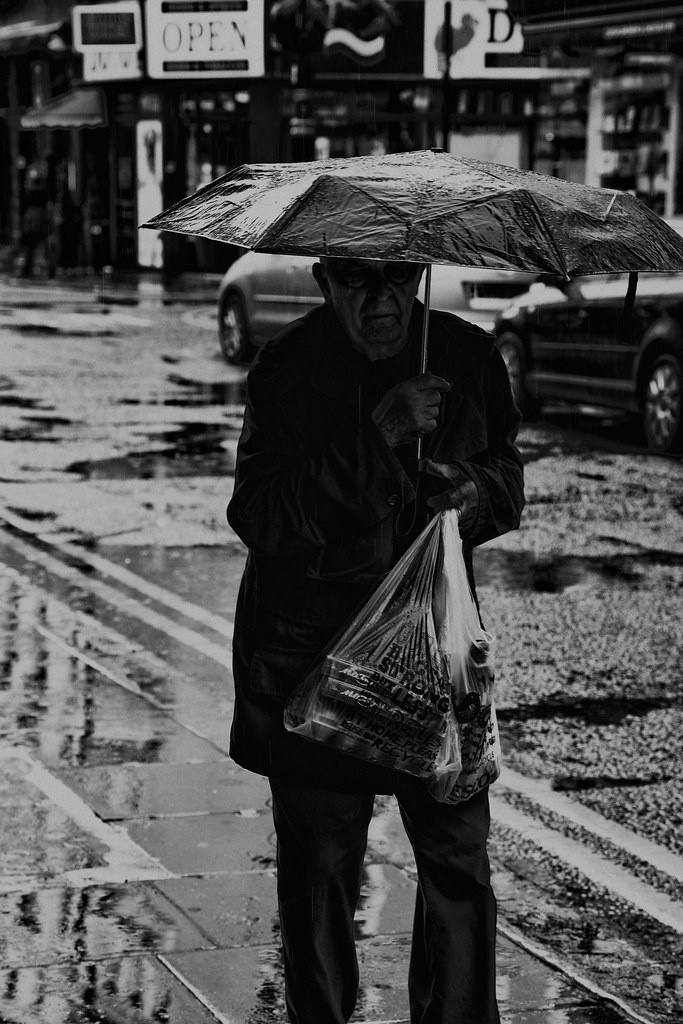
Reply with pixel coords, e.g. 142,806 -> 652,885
215,250 -> 537,370
493,220 -> 683,458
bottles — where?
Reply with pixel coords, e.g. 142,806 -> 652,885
452,639 -> 493,790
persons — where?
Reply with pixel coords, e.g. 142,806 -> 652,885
226,254 -> 528,1024
0,154 -> 122,280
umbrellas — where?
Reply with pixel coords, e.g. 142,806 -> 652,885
133,147 -> 683,471
